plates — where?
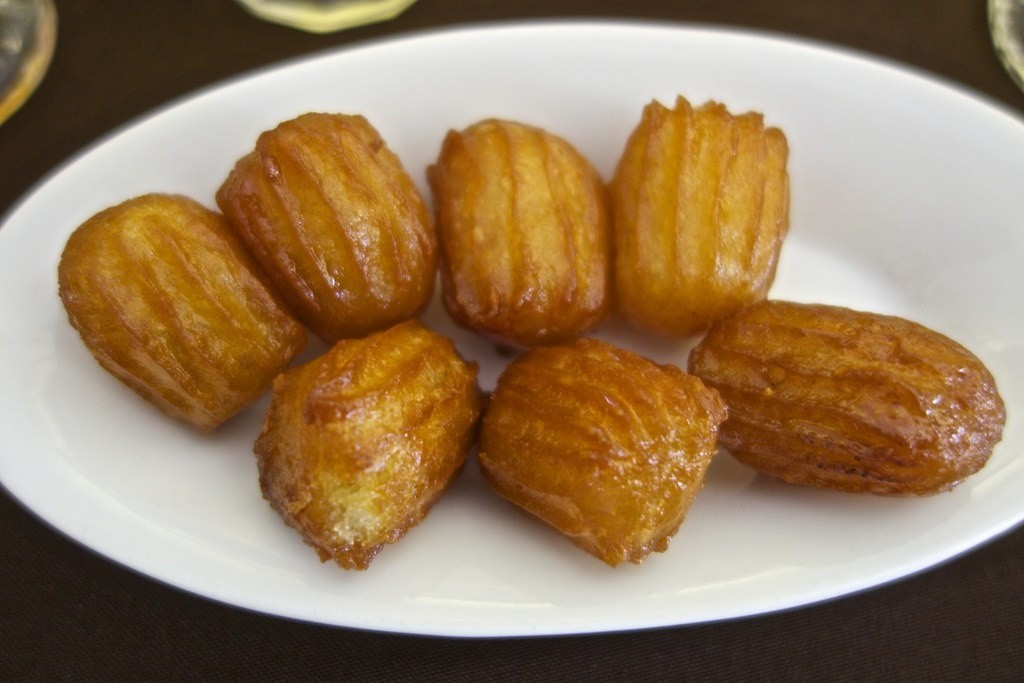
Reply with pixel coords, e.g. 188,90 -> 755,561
0,21 -> 1024,638
